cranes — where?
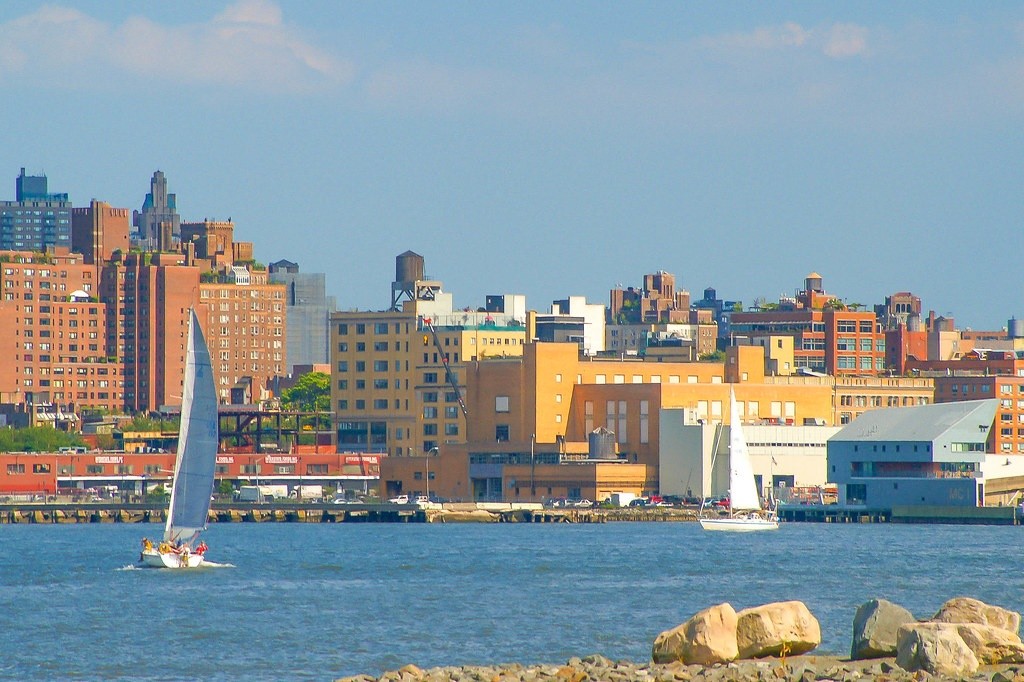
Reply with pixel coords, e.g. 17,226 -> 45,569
420,317 -> 467,422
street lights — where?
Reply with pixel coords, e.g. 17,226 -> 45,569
255,453 -> 270,501
530,433 -> 535,501
697,419 -> 706,502
426,446 -> 439,500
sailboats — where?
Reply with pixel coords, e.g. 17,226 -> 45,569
142,307 -> 218,568
696,376 -> 779,532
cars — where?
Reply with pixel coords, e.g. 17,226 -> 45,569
573,499 -> 594,508
387,494 -> 433,506
553,499 -> 574,507
334,498 -> 364,504
605,494 -> 730,511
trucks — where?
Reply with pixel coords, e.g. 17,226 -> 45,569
288,485 -> 323,502
229,485 -> 287,502
